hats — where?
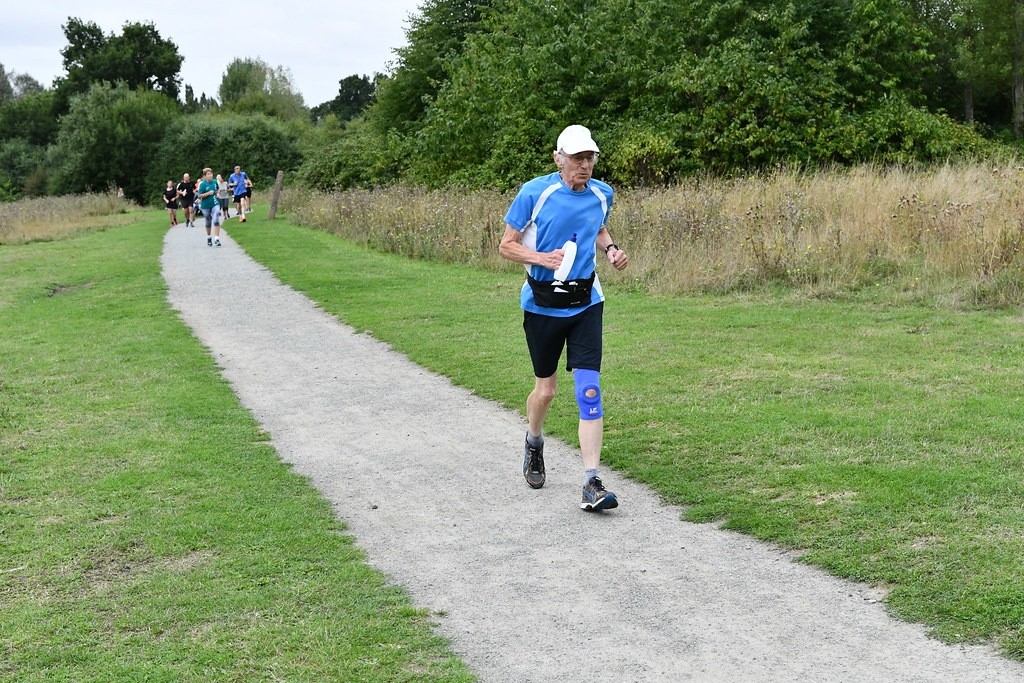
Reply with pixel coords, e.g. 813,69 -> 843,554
557,125 -> 599,154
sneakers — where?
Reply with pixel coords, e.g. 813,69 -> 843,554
523,433 -> 545,488
208,238 -> 212,246
215,240 -> 221,247
581,477 -> 618,510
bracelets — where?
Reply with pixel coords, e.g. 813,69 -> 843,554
604,245 -> 621,254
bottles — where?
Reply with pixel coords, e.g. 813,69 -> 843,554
553,233 -> 577,283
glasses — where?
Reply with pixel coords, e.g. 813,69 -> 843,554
559,152 -> 599,164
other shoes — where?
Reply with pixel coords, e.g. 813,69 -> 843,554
186,220 -> 189,227
190,222 -> 194,227
227,213 -> 230,219
240,216 -> 246,222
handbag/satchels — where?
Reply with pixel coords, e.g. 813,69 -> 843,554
528,270 -> 595,309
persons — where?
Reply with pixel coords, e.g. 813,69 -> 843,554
162,165 -> 254,249
500,125 -> 627,512
